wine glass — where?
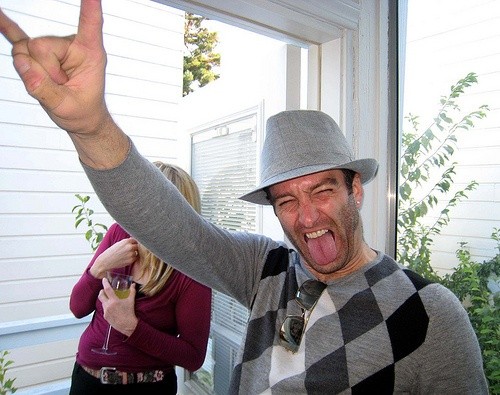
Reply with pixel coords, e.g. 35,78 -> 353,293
91,271 -> 133,355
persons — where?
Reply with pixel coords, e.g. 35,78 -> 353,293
0,1 -> 500,395
70,161 -> 212,394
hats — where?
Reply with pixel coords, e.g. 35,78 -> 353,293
239,110 -> 379,205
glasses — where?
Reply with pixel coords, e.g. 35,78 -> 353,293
277,279 -> 329,353
